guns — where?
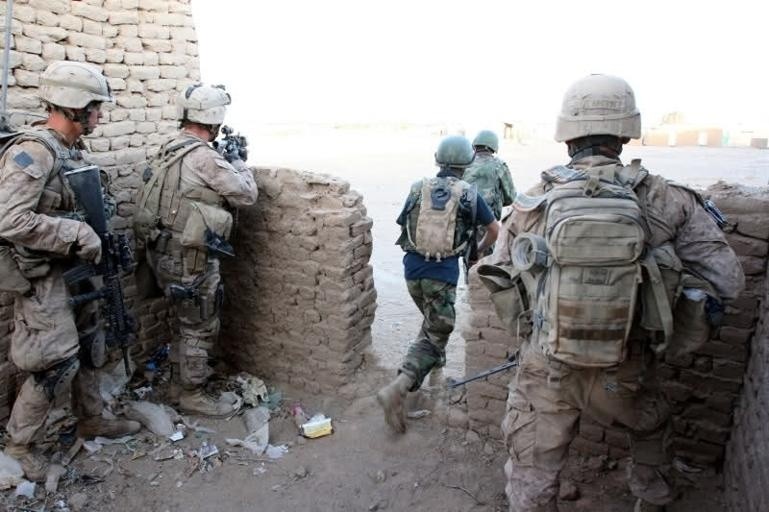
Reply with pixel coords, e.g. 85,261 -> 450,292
62,165 -> 137,377
213,125 -> 248,154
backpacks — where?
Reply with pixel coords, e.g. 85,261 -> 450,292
416,177 -> 466,259
528,162 -> 649,371
466,159 -> 503,207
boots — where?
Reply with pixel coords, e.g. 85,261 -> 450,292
4,438 -> 68,482
77,414 -> 141,441
377,372 -> 413,433
171,383 -> 235,419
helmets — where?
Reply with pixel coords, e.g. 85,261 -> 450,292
37,60 -> 113,110
175,82 -> 230,125
472,130 -> 497,152
554,73 -> 642,142
434,135 -> 475,168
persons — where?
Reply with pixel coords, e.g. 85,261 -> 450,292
0,61 -> 141,483
134,83 -> 259,419
377,134 -> 500,434
491,73 -> 747,512
462,130 -> 518,285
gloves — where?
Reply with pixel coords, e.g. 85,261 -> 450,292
223,149 -> 241,162
76,221 -> 103,266
